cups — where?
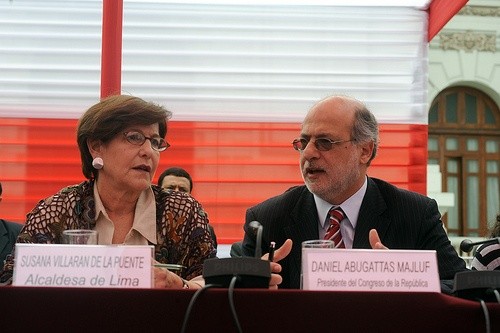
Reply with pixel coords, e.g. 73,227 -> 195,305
63,230 -> 99,246
300,239 -> 334,290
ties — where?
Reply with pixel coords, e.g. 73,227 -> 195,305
322,205 -> 347,248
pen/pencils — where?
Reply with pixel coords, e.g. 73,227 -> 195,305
268,242 -> 275,262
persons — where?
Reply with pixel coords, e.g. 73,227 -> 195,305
0,92 -> 217,289
158,167 -> 193,195
241,95 -> 490,301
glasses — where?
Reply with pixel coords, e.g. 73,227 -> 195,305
118,129 -> 171,151
290,138 -> 352,151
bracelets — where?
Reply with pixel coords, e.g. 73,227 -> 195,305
182,278 -> 190,289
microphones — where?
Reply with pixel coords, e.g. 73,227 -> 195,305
452,237 -> 500,295
202,221 -> 270,289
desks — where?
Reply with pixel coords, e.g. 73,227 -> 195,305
0,285 -> 500,333
